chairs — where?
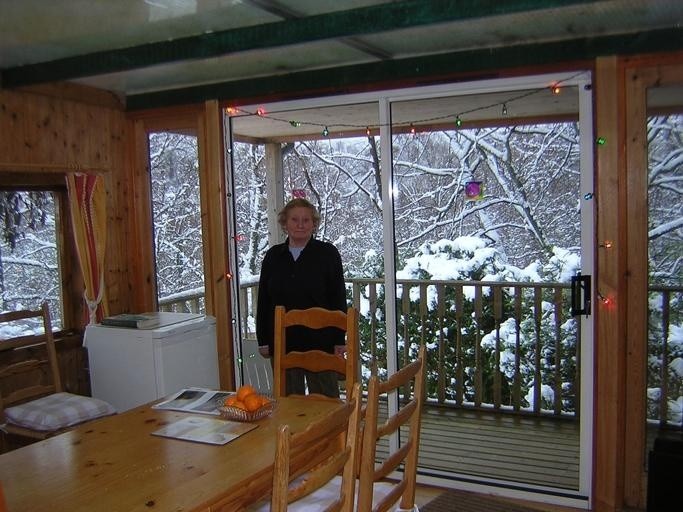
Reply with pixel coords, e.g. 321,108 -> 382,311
270,305 -> 358,402
255,383 -> 361,512
0,302 -> 98,438
282,344 -> 427,512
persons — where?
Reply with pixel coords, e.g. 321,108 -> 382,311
256,198 -> 348,396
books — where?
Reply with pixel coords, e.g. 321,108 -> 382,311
149,415 -> 260,445
101,311 -> 161,329
151,387 -> 234,417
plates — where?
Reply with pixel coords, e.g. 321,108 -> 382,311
215,392 -> 277,422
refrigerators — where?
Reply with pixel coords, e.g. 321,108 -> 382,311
86,311 -> 220,414
